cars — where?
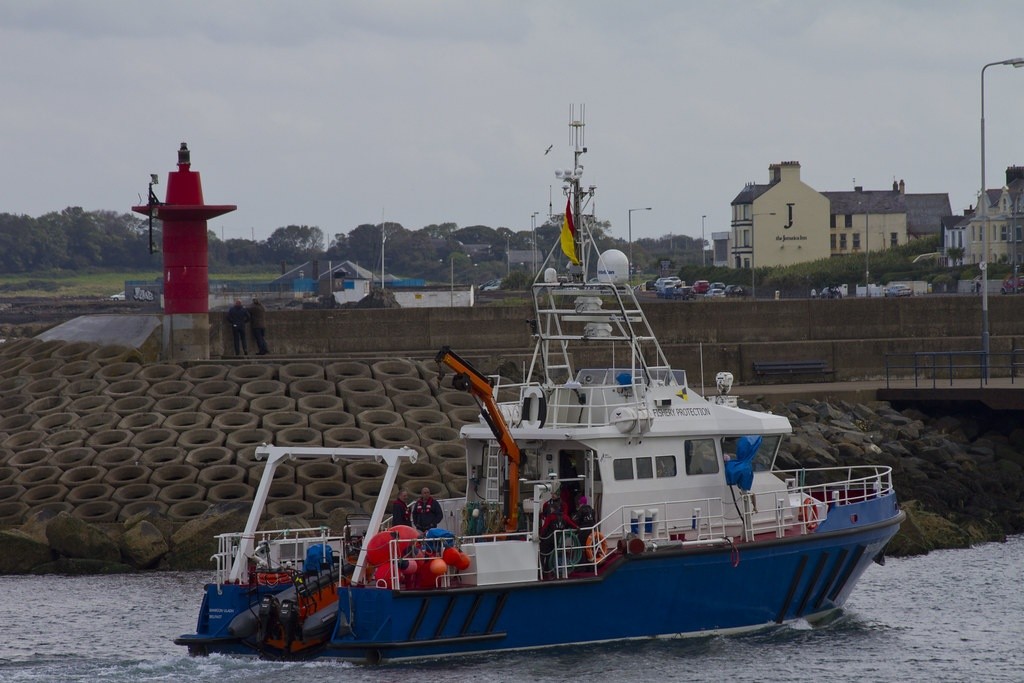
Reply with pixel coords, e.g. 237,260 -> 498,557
885,284 -> 914,297
631,274 -> 746,301
819,286 -> 842,299
999,271 -> 1024,296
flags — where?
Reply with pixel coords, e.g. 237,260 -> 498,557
560,199 -> 579,264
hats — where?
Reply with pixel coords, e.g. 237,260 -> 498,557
579,497 -> 587,505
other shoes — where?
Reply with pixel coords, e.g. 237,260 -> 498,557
244,352 -> 249,355
236,352 -> 239,355
256,351 -> 265,355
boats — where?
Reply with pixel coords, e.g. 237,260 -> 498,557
165,98 -> 911,671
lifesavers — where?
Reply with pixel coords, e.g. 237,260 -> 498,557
586,531 -> 607,564
258,573 -> 290,584
803,498 -> 818,530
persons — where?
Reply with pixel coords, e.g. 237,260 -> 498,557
226,300 -> 251,356
543,495 -> 580,572
250,298 -> 269,355
572,496 -> 594,547
387,489 -> 411,527
412,487 -> 443,533
811,288 -> 816,299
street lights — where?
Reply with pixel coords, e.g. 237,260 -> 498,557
531,211 -> 540,278
628,207 -> 652,282
701,215 -> 707,268
979,56 -> 1024,382
